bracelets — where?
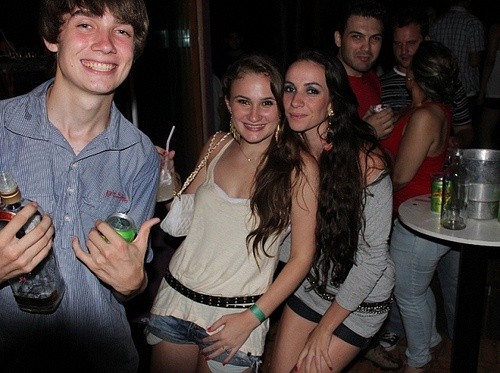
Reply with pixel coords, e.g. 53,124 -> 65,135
249,304 -> 266,323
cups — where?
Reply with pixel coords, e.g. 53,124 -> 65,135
156,152 -> 175,202
370,104 -> 390,140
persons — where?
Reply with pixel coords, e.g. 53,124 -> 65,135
378,16 -> 469,341
333,4 -> 403,371
381,40 -> 456,373
0,0 -> 159,373
428,1 -> 486,105
269,50 -> 397,373
147,58 -> 321,373
471,19 -> 500,149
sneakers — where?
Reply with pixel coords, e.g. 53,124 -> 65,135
380,330 -> 400,352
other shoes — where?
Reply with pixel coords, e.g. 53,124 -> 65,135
361,344 -> 403,371
404,361 -> 432,373
400,341 -> 443,364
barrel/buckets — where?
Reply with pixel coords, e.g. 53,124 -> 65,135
455,148 -> 500,221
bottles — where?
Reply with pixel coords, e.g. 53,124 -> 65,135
0,170 -> 65,314
441,147 -> 467,229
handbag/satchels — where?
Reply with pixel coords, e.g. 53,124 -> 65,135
160,131 -> 231,237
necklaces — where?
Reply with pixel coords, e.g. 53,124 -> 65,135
238,144 -> 262,162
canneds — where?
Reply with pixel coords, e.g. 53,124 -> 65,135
431,177 -> 451,214
100,213 -> 136,244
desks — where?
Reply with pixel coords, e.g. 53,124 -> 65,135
399,193 -> 500,373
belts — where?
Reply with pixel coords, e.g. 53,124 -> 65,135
162,267 -> 264,308
